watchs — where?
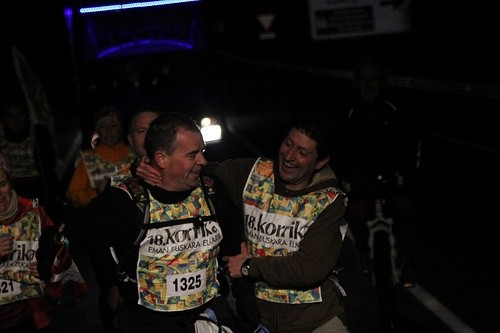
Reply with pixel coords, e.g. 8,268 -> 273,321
240,258 -> 251,278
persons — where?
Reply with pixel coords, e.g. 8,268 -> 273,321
0,94 -> 350,333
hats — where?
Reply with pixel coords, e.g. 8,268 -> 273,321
94,107 -> 122,131
